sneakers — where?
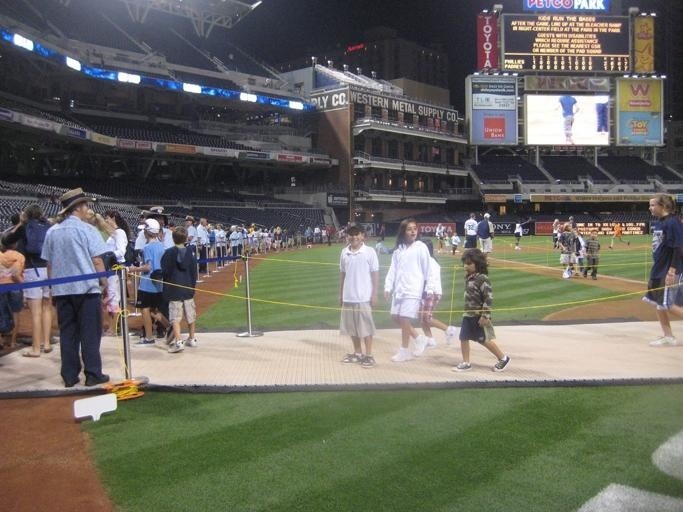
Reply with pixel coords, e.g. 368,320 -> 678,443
343,354 -> 364,363
186,338 -> 197,348
493,356 -> 511,372
451,362 -> 472,372
363,356 -> 376,368
591,273 -> 597,280
428,339 -> 437,347
414,335 -> 428,356
166,326 -> 175,344
65,377 -> 80,386
392,349 -> 412,361
85,374 -> 109,386
135,338 -> 155,345
168,341 -> 183,352
445,326 -> 458,345
583,271 -> 587,278
649,335 -> 679,347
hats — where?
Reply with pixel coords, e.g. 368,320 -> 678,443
184,216 -> 194,221
137,218 -> 160,233
347,222 -> 363,232
484,213 -> 491,218
57,188 -> 96,215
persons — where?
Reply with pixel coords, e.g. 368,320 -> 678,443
642,194 -> 683,347
336,228 -> 346,242
476,213 -> 494,266
86,209 -> 116,239
129,218 -> 176,347
592,95 -> 610,136
41,188 -> 113,388
3,207 -> 22,236
422,240 -> 456,347
215,225 -> 225,268
451,232 -> 461,255
383,219 -> 430,362
608,222 -> 630,250
435,223 -> 445,253
556,224 -> 563,249
552,219 -> 560,249
572,230 -> 587,275
447,226 -> 452,241
103,252 -> 122,336
379,223 -> 385,241
10,203 -> 53,357
339,226 -> 380,368
166,221 -> 176,231
583,230 -> 600,280
375,237 -> 394,256
229,226 -> 239,256
135,207 -> 176,339
196,218 -> 210,273
514,217 -> 532,250
238,227 -> 243,255
0,233 -> 26,350
183,216 -> 197,252
160,226 -> 197,353
452,249 -> 511,372
0,221 -> 5,232
443,234 -> 450,249
207,224 -> 215,274
224,224 -> 238,255
569,216 -> 573,223
248,223 -> 336,257
558,223 -> 575,279
241,224 -> 247,255
465,213 -> 478,250
554,95 -> 580,145
105,210 -> 131,299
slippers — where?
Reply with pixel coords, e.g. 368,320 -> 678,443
44,346 -> 52,354
23,351 -> 40,357
